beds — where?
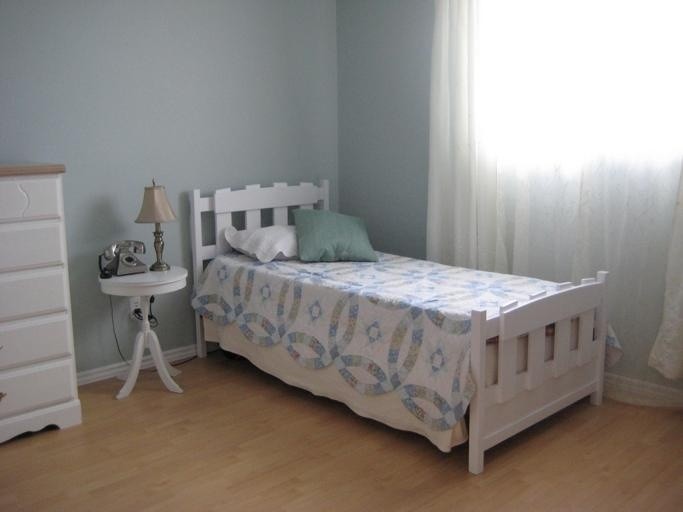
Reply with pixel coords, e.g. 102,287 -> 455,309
187,178 -> 612,476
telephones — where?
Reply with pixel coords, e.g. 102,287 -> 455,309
104,240 -> 148,276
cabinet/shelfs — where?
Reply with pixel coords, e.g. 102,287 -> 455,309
0,162 -> 82,445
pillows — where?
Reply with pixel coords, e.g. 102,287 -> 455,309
222,206 -> 383,266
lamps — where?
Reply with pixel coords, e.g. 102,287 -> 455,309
134,179 -> 180,270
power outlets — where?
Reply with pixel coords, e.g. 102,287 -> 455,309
128,296 -> 140,320
